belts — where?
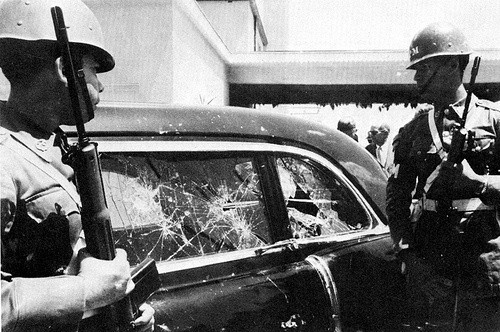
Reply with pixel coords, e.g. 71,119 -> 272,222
417,199 -> 495,211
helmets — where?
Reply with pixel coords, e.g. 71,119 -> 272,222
407,25 -> 472,69
0,0 -> 115,73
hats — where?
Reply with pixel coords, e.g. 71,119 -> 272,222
338,121 -> 357,135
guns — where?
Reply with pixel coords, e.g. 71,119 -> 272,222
50,4 -> 163,332
407,57 -> 481,314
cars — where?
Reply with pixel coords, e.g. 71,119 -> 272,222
0,101 -> 409,332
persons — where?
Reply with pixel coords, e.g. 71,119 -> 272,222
0,1 -> 157,332
385,28 -> 499,332
338,117 -> 395,176
